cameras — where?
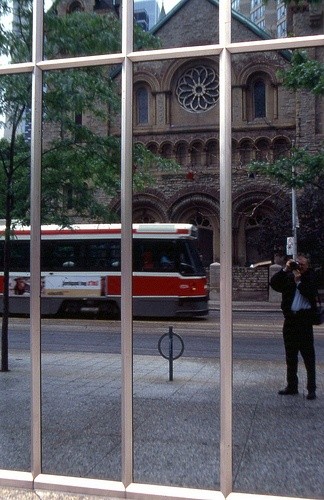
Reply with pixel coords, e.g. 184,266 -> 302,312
290,260 -> 299,270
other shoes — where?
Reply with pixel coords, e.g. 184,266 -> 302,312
278,389 -> 298,396
306,392 -> 316,399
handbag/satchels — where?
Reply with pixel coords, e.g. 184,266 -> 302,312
313,306 -> 324,325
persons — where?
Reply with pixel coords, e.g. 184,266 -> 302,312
160,250 -> 174,271
13,277 -> 30,296
210,256 -> 221,266
270,255 -> 318,400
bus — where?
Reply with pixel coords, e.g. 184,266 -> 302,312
0,223 -> 209,320
